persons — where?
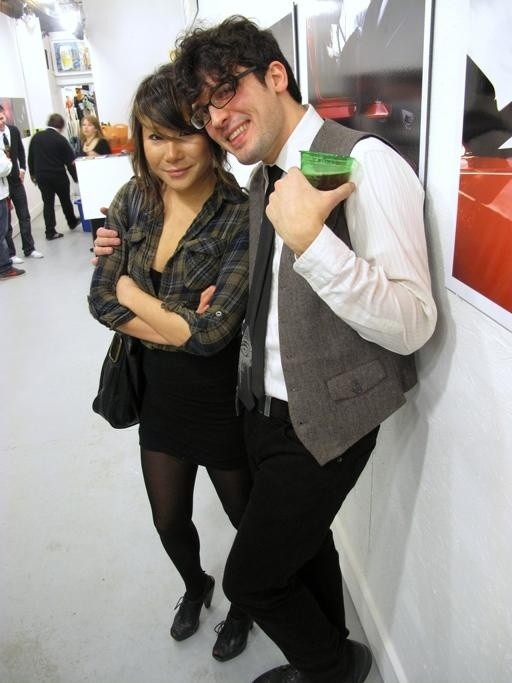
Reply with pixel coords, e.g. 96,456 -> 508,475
63,85 -> 96,120
88,63 -> 254,665
28,113 -> 81,240
1,145 -> 26,278
91,13 -> 436,683
72,115 -> 112,165
1,104 -> 42,263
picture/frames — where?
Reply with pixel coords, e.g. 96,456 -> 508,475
50,39 -> 92,76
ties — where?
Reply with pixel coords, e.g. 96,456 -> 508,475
2,133 -> 9,148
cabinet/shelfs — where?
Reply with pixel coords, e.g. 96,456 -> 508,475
74,152 -> 140,252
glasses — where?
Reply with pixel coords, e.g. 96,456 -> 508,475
189,66 -> 257,130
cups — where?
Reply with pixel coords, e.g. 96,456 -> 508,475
300,152 -> 353,230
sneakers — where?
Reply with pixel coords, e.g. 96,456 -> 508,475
46,232 -> 64,240
27,251 -> 44,259
71,218 -> 81,230
10,256 -> 24,264
0,267 -> 25,280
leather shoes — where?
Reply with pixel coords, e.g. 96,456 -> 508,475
252,640 -> 372,683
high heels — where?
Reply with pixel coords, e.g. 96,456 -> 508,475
212,614 -> 253,662
170,575 -> 215,641
